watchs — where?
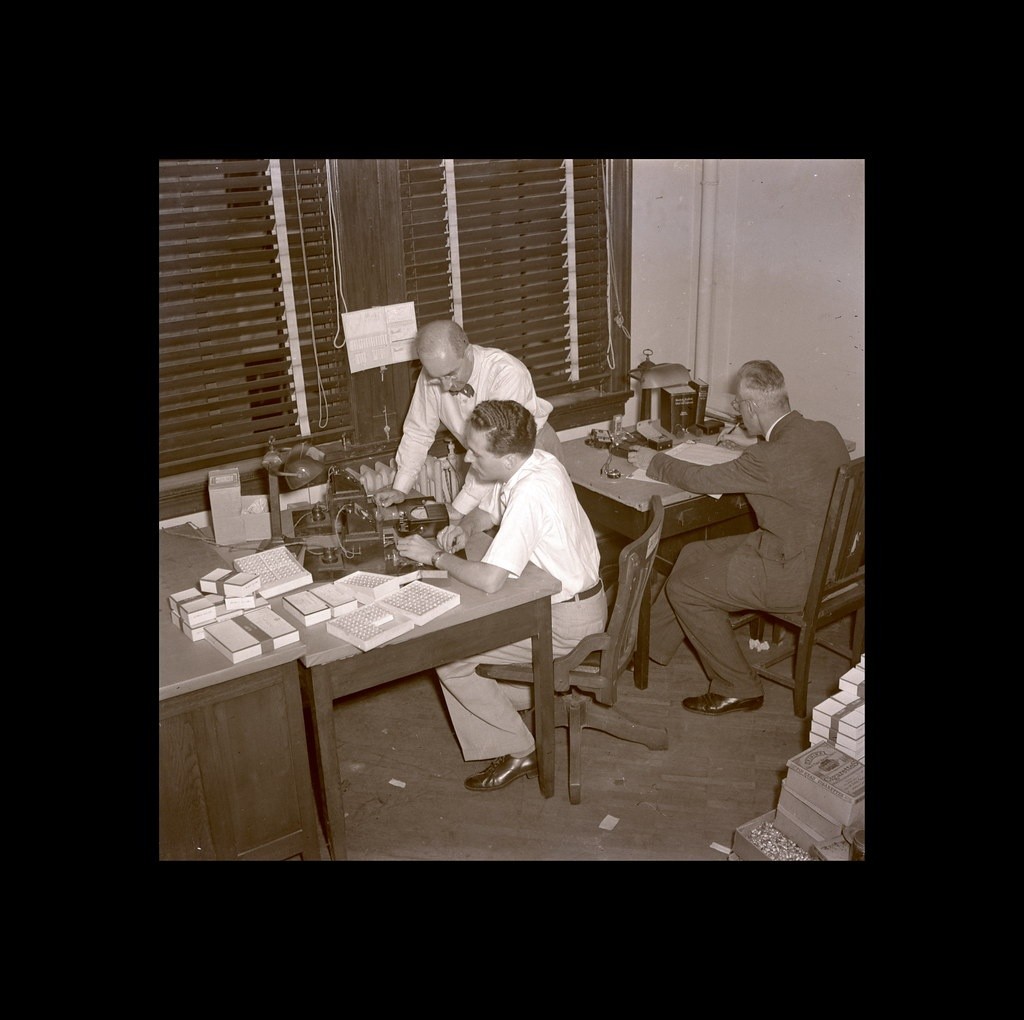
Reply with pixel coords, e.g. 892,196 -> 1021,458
430,548 -> 446,569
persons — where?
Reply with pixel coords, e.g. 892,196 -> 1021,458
626,360 -> 856,716
396,399 -> 609,793
373,320 -> 566,519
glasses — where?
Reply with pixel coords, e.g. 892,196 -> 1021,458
731,397 -> 760,412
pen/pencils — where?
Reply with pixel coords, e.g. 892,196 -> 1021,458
715,422 -> 740,447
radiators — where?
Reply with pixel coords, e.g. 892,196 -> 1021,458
345,436 -> 460,502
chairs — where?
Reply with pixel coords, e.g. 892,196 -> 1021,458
475,494 -> 669,806
730,455 -> 865,719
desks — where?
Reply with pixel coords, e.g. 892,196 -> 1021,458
199,484 -> 563,861
160,524 -> 324,861
559,415 -> 857,692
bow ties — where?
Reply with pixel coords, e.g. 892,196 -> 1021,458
449,384 -> 476,399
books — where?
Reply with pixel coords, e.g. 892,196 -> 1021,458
660,378 -> 710,433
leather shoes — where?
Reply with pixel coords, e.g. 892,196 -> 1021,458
463,753 -> 539,792
517,691 -> 564,717
682,691 -> 765,715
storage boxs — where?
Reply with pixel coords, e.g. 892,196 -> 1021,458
241,495 -> 271,541
210,517 -> 247,548
208,468 -> 244,519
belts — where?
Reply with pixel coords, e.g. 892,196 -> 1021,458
560,579 -> 602,603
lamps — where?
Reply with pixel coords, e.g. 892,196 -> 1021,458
629,348 -> 692,450
255,435 -> 327,556
